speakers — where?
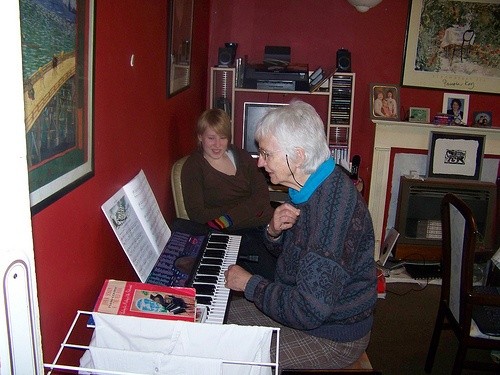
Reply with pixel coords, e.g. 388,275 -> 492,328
218,47 -> 233,67
336,48 -> 351,72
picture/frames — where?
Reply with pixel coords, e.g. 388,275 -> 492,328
399,0 -> 500,96
442,93 -> 471,126
472,111 -> 492,129
408,107 -> 431,124
166,0 -> 194,99
425,130 -> 487,181
19,0 -> 96,218
368,81 -> 401,121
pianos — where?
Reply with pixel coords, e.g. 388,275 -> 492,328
144,217 -> 244,325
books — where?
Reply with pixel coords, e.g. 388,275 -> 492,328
101,169 -> 172,284
380,227 -> 399,266
86,280 -> 207,327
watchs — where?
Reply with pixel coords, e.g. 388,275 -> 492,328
267,221 -> 282,239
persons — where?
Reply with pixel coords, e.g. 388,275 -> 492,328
223,99 -> 377,372
446,99 -> 463,119
181,108 -> 280,268
374,91 -> 397,117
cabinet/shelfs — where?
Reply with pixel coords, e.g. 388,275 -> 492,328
210,66 -> 357,162
42,310 -> 281,375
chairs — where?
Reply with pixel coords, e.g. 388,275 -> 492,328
422,193 -> 500,375
171,155 -> 190,220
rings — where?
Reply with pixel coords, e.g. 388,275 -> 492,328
280,225 -> 282,230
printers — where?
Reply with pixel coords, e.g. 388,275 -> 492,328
243,45 -> 310,92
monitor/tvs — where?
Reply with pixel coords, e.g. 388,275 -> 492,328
242,102 -> 289,158
